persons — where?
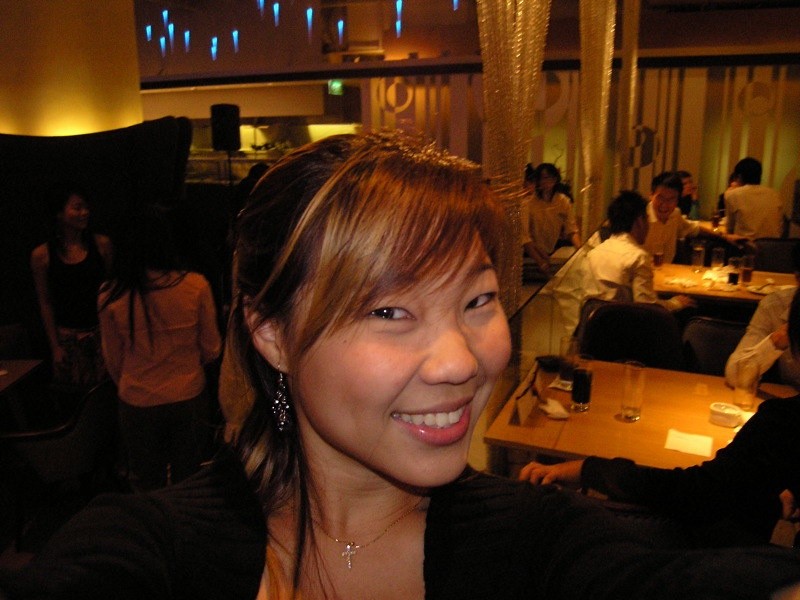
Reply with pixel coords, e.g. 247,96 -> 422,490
95,210 -> 222,495
30,182 -> 118,486
0,124 -> 800,600
479,157 -> 800,519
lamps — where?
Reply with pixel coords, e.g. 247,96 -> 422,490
210,104 -> 242,185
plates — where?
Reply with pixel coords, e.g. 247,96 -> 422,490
746,285 -> 795,295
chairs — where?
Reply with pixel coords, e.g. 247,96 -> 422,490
0,380 -> 118,552
583,303 -> 749,372
755,237 -> 800,272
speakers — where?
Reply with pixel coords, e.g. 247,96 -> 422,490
211,104 -> 241,152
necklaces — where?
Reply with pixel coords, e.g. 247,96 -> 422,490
285,489 -> 427,569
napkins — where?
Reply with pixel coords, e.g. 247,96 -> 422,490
665,429 -> 712,457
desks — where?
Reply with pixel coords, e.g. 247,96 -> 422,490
484,358 -> 769,479
653,263 -> 800,302
0,359 -> 44,428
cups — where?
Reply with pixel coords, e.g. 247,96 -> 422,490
621,360 -> 645,421
570,353 -> 594,411
728,254 -> 754,285
732,358 -> 760,411
653,242 -> 665,268
711,209 -> 720,230
558,336 -> 581,384
711,248 -> 725,268
691,239 -> 706,274
